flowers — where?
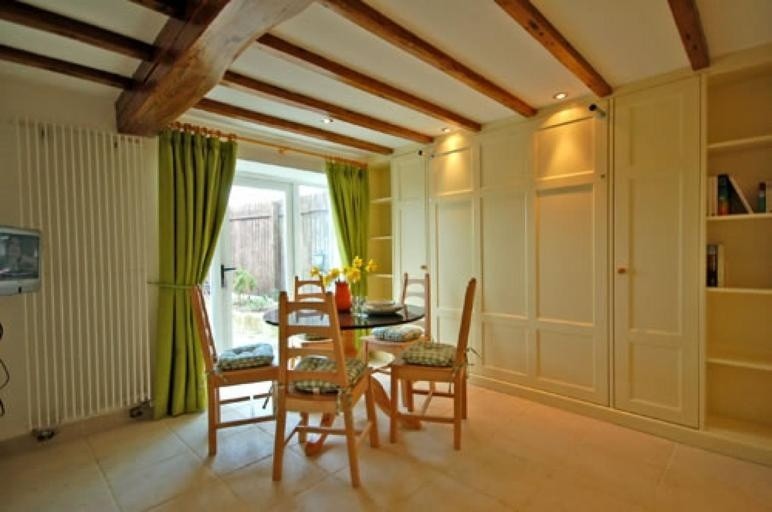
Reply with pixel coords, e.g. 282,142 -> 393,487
309,255 -> 379,287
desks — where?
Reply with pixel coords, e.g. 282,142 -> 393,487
264,305 -> 425,457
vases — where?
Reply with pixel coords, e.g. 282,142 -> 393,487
334,281 -> 353,312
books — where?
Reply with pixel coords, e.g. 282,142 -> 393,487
707,173 -> 754,216
706,242 -> 726,288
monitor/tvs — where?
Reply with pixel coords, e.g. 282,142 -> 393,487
0,225 -> 41,296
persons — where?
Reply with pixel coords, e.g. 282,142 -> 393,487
1,235 -> 36,276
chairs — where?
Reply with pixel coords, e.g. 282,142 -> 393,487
272,291 -> 380,489
294,275 -> 326,302
388,275 -> 479,450
362,270 -> 430,409
192,285 -> 280,456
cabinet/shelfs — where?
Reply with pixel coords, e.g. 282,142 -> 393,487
613,73 -> 701,443
390,148 -> 430,336
701,43 -> 771,467
